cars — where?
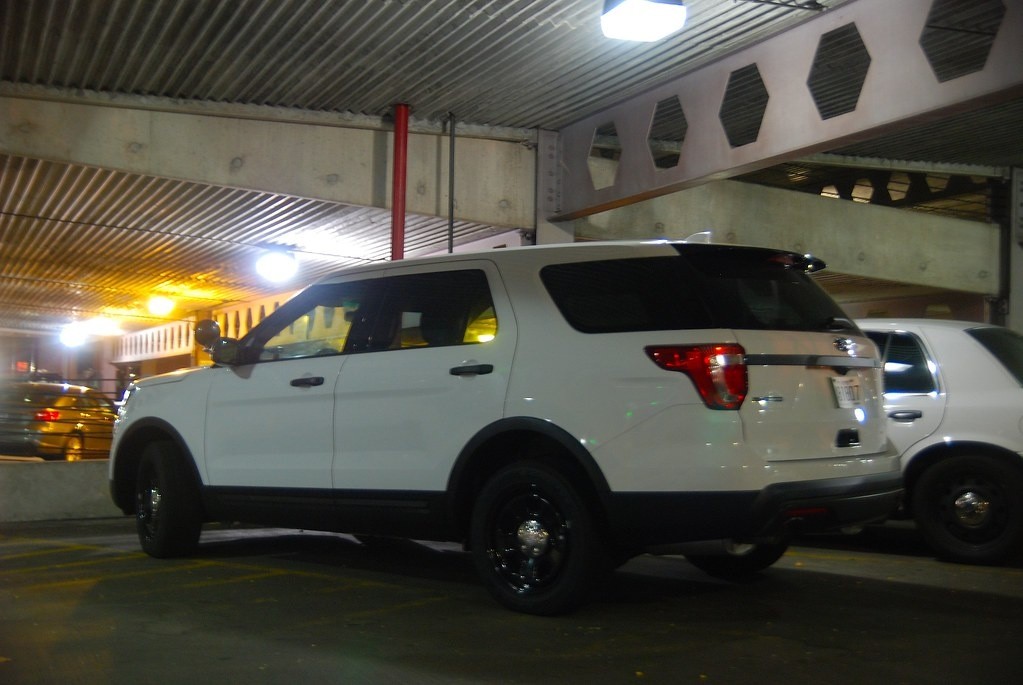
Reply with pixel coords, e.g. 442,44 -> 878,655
841,318 -> 1023,564
0,378 -> 116,463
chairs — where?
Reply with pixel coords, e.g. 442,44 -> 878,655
352,302 -> 401,351
420,299 -> 471,346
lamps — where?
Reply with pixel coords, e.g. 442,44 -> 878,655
602,0 -> 827,42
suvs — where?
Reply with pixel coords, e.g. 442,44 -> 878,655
108,232 -> 905,612
